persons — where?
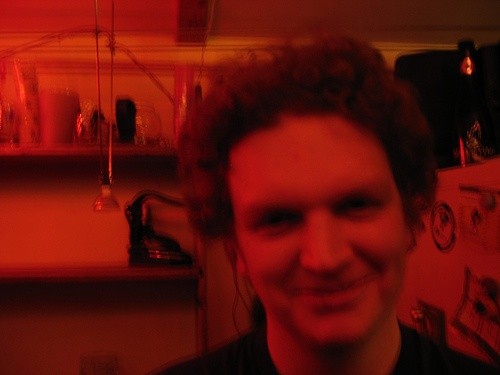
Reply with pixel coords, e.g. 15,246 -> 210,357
156,37 -> 499,375
